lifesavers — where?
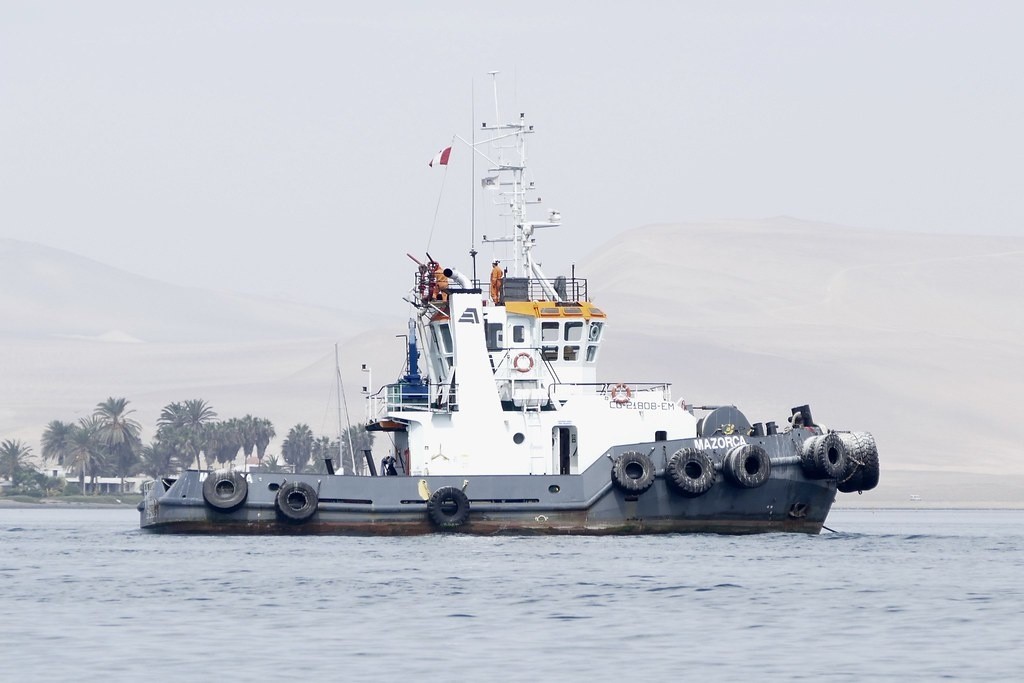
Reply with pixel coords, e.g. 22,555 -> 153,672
514,353 -> 534,373
202,470 -> 248,512
800,428 -> 880,496
724,445 -> 773,490
611,450 -> 655,496
666,447 -> 717,497
612,384 -> 631,404
273,481 -> 319,523
427,487 -> 470,528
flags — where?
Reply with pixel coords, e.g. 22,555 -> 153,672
429,146 -> 451,167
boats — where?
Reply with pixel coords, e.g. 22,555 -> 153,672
138,70 -> 870,539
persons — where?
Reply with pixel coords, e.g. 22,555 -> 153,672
432,266 -> 449,302
490,260 -> 503,306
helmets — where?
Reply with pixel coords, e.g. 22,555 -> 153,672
492,260 -> 497,263
